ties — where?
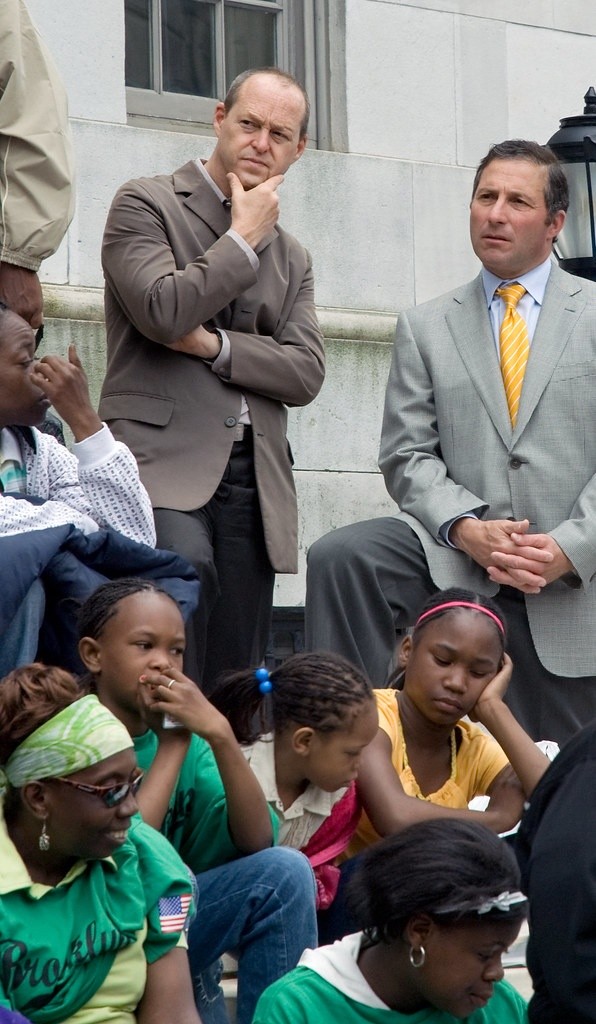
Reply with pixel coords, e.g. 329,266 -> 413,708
495,285 -> 531,430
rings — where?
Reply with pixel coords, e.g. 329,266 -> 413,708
166,679 -> 177,689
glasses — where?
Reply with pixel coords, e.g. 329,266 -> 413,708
40,768 -> 144,808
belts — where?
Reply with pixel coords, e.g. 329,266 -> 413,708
234,423 -> 252,442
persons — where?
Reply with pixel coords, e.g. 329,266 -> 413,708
0,574 -> 596,1024
304,140 -> 596,750
0,0 -> 77,350
0,306 -> 158,676
95,64 -> 327,706
